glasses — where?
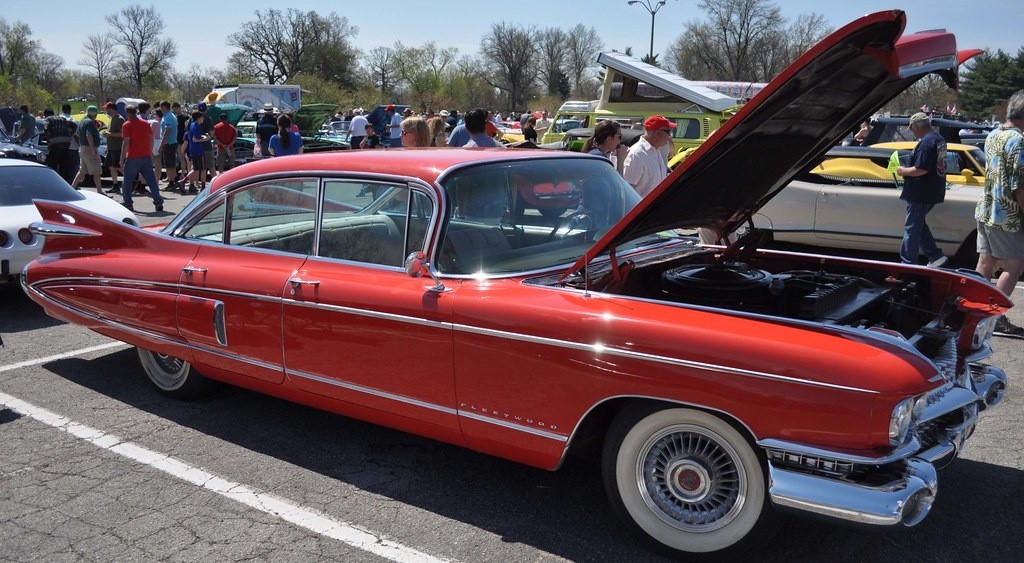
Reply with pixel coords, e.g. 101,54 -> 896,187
612,134 -> 622,139
659,129 -> 671,134
402,130 -> 412,135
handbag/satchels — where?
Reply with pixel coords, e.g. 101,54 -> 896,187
69,133 -> 79,150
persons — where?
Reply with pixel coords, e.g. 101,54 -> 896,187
897,112 -> 948,267
254,103 -> 304,157
11,105 -> 36,144
975,89 -> 1024,339
581,120 -> 629,176
119,104 -> 164,212
326,105 -> 552,220
37,100 -> 238,196
623,114 -> 676,198
853,118 -> 874,139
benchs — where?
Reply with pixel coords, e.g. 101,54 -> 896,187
230,215 -> 518,274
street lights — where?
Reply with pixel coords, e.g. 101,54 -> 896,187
628,0 -> 667,65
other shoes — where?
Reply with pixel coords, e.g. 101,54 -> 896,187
106,187 -> 120,193
927,256 -> 947,268
135,181 -> 150,193
123,205 -> 133,210
156,204 -> 163,211
993,315 -> 1024,339
189,186 -> 198,193
178,180 -> 186,192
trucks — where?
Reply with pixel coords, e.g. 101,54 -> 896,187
204,83 -> 314,116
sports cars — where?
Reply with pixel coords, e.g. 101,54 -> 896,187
21,10 -> 1016,563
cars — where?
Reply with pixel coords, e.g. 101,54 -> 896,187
175,100 -> 409,172
496,119 -> 549,145
0,158 -> 140,279
734,117 -> 1005,279
0,108 -> 114,178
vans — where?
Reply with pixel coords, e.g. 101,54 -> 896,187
538,50 -> 771,171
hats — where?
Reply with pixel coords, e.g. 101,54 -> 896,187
907,112 -> 928,129
198,102 -> 206,108
643,115 -> 677,131
88,105 -> 98,112
257,103 -> 280,114
385,105 -> 394,111
220,113 -> 227,119
102,102 -> 116,109
